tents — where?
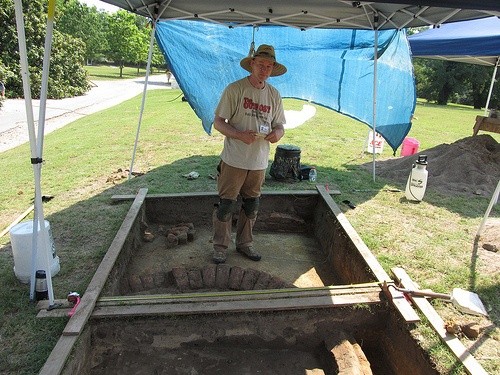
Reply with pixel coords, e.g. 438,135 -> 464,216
407,16 -> 500,116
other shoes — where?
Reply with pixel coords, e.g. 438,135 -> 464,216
213,249 -> 227,263
236,246 -> 262,261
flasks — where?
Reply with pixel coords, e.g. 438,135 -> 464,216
35,270 -> 48,298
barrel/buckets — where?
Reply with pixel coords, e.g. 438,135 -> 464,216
401,137 -> 420,156
10,219 -> 60,284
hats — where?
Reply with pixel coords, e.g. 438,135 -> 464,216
240,44 -> 287,77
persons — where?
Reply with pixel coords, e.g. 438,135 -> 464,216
210,44 -> 287,263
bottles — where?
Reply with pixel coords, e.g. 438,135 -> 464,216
308,167 -> 317,182
405,155 -> 429,201
